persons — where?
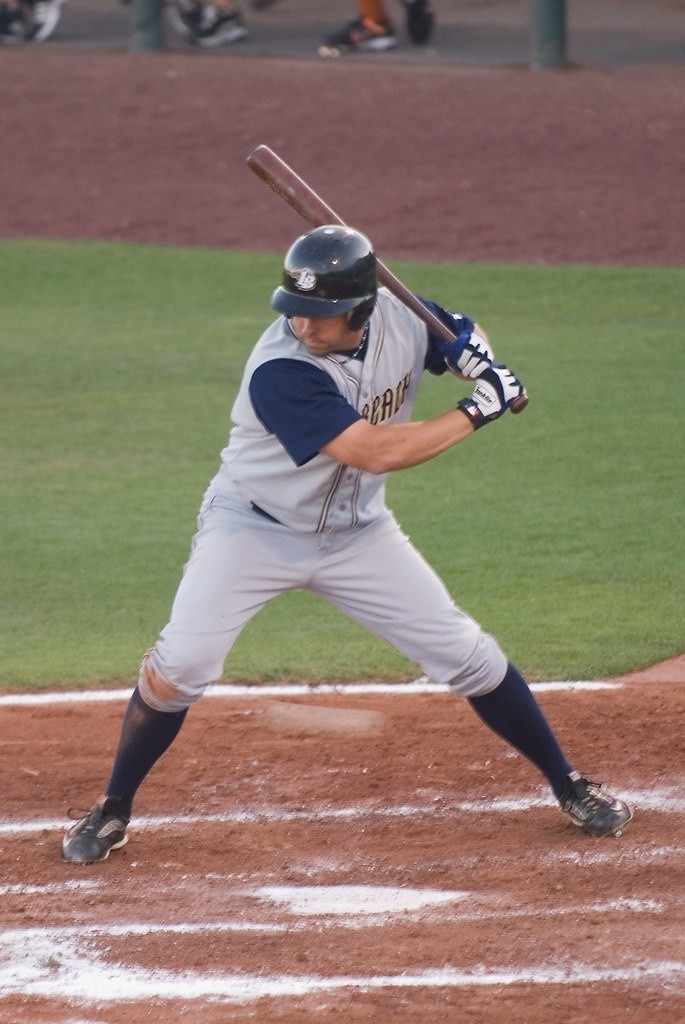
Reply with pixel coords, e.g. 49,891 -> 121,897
0,0 -> 585,71
61,225 -> 633,865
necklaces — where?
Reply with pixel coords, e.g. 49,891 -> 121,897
338,322 -> 367,366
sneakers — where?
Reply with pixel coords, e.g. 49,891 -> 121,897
0,0 -> 64,44
324,16 -> 397,52
166,0 -> 248,47
557,770 -> 634,840
404,0 -> 435,45
61,794 -> 129,865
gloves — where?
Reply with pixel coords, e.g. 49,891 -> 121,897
440,329 -> 495,380
457,365 -> 527,432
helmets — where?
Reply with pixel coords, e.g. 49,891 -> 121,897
270,225 -> 378,331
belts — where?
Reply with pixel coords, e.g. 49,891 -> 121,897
251,501 -> 281,524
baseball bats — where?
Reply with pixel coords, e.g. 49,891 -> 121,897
244,146 -> 529,415
